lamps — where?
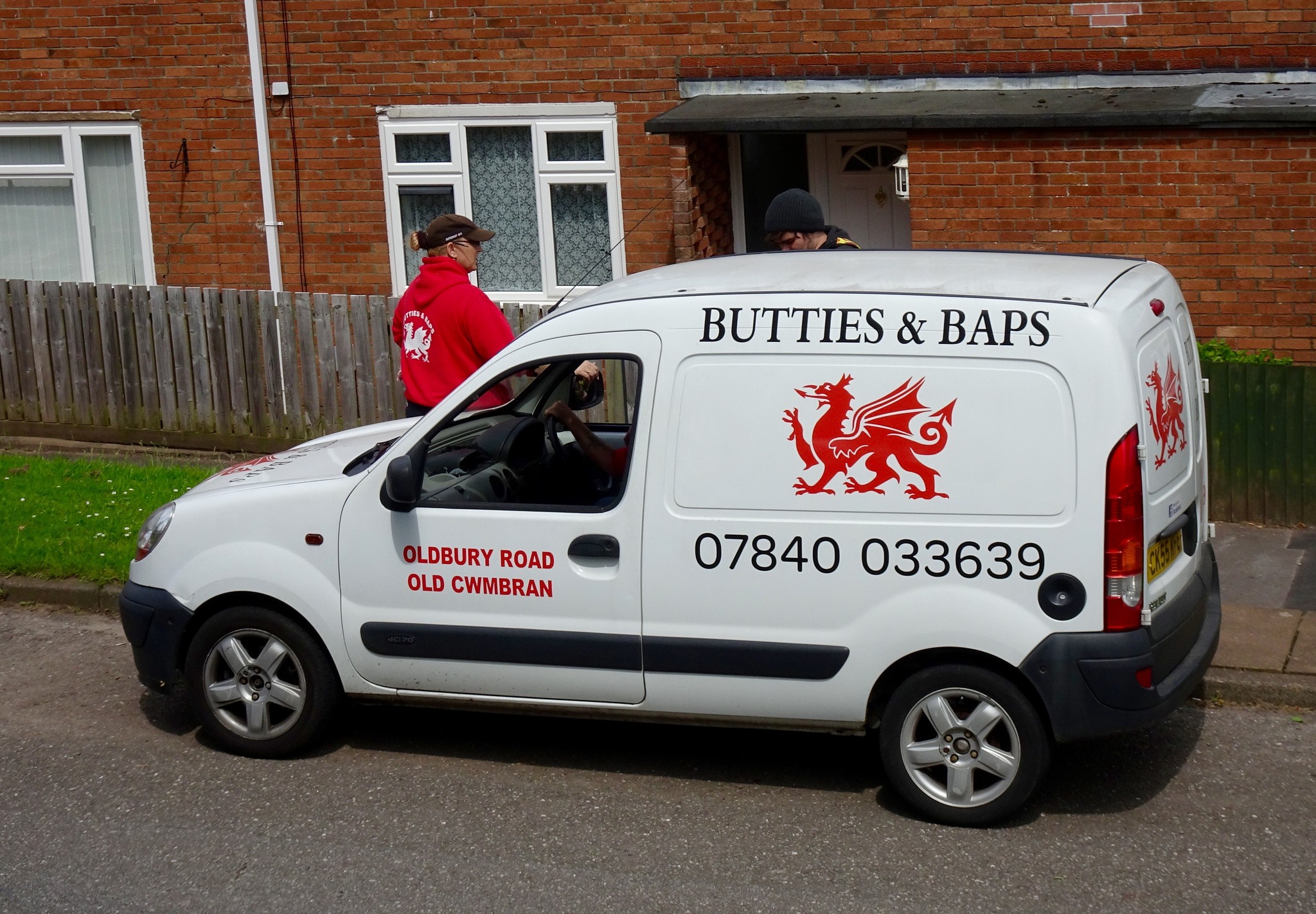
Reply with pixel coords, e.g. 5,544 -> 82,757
893,148 -> 910,203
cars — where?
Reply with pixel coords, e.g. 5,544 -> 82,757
121,249 -> 1226,826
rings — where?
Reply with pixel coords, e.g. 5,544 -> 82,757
588,370 -> 591,374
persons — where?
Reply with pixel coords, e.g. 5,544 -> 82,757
391,213 -> 600,419
763,188 -> 862,251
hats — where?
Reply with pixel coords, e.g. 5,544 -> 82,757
424,214 -> 496,250
764,189 -> 826,231
